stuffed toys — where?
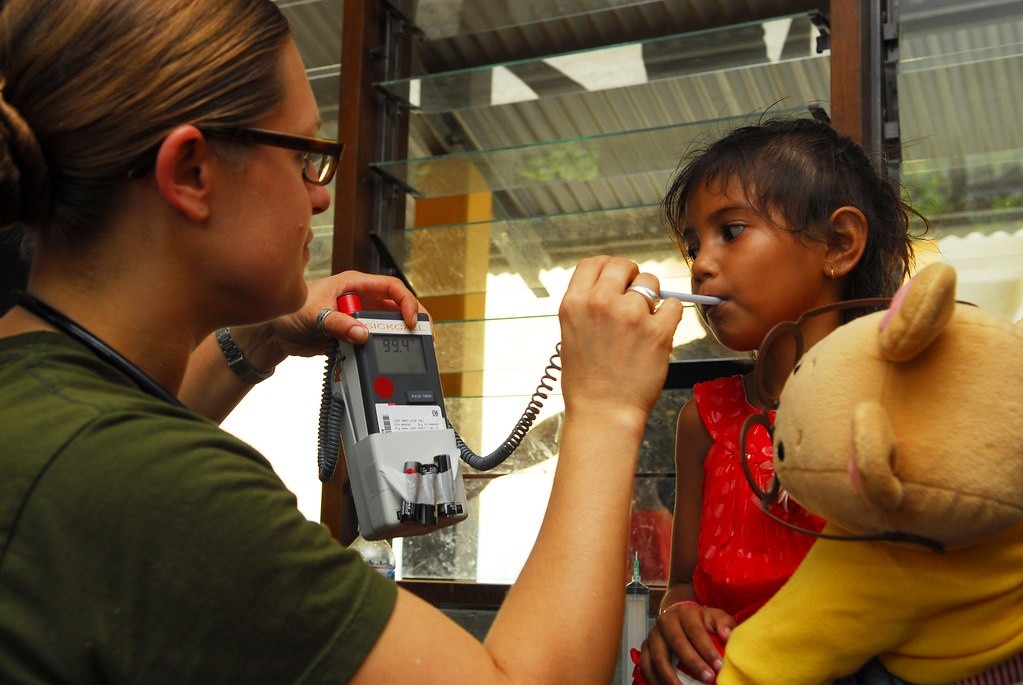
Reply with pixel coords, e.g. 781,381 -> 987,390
716,263 -> 1023,685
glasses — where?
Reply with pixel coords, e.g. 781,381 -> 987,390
127,121 -> 345,185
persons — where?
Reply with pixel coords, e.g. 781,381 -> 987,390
0,0 -> 684,685
462,307 -> 758,586
630,116 -> 912,685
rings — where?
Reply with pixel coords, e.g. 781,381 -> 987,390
625,285 -> 660,314
316,308 -> 334,338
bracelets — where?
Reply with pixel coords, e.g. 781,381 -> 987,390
656,600 -> 700,623
216,327 -> 275,384
